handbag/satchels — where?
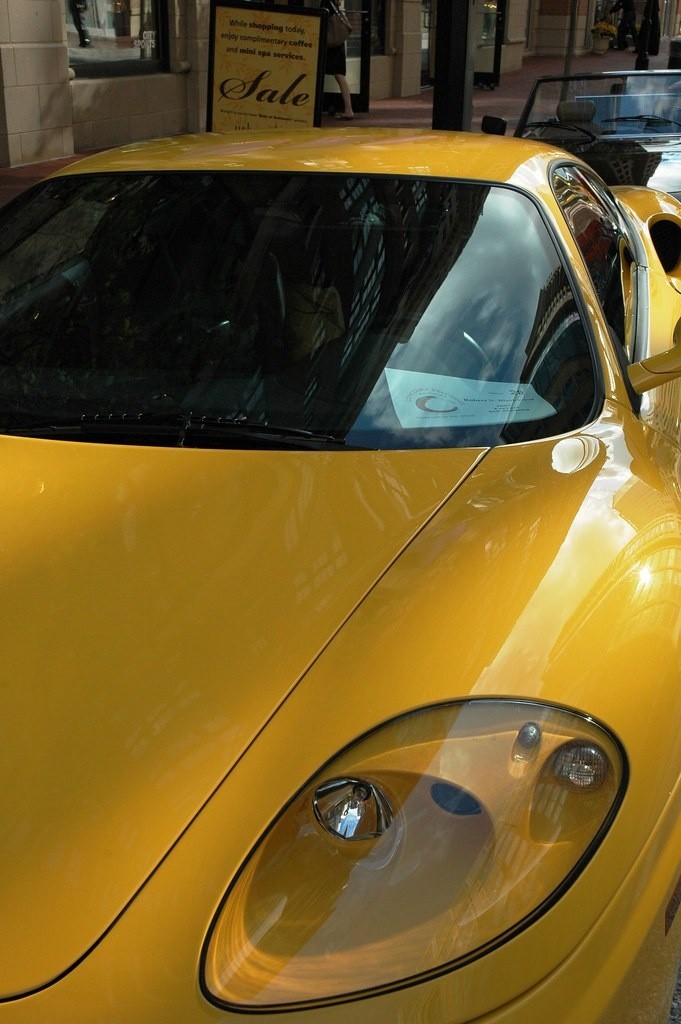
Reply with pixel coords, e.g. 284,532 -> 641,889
321,0 -> 353,50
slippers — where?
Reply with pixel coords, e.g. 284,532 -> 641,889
333,114 -> 353,120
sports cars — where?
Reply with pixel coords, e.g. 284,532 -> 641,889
1,127 -> 681,1024
479,68 -> 681,201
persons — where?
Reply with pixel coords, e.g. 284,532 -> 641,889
633,0 -> 661,56
320,0 -> 360,119
608,0 -> 641,53
